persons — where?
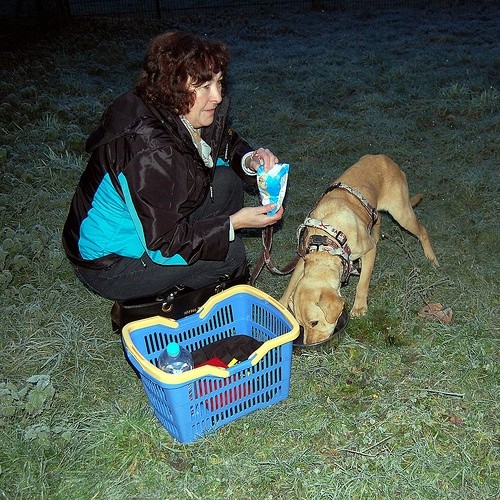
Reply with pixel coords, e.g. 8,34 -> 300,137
62,31 -> 284,335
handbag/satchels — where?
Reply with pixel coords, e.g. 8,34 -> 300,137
120,263 -> 250,378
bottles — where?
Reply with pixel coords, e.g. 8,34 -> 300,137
158,342 -> 197,415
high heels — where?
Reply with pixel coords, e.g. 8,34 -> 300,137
111,300 -> 121,335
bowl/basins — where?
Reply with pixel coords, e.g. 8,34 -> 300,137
293,302 -> 350,357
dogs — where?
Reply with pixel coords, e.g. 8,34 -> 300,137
278,154 -> 440,345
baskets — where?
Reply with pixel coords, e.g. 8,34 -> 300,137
122,285 -> 300,444
